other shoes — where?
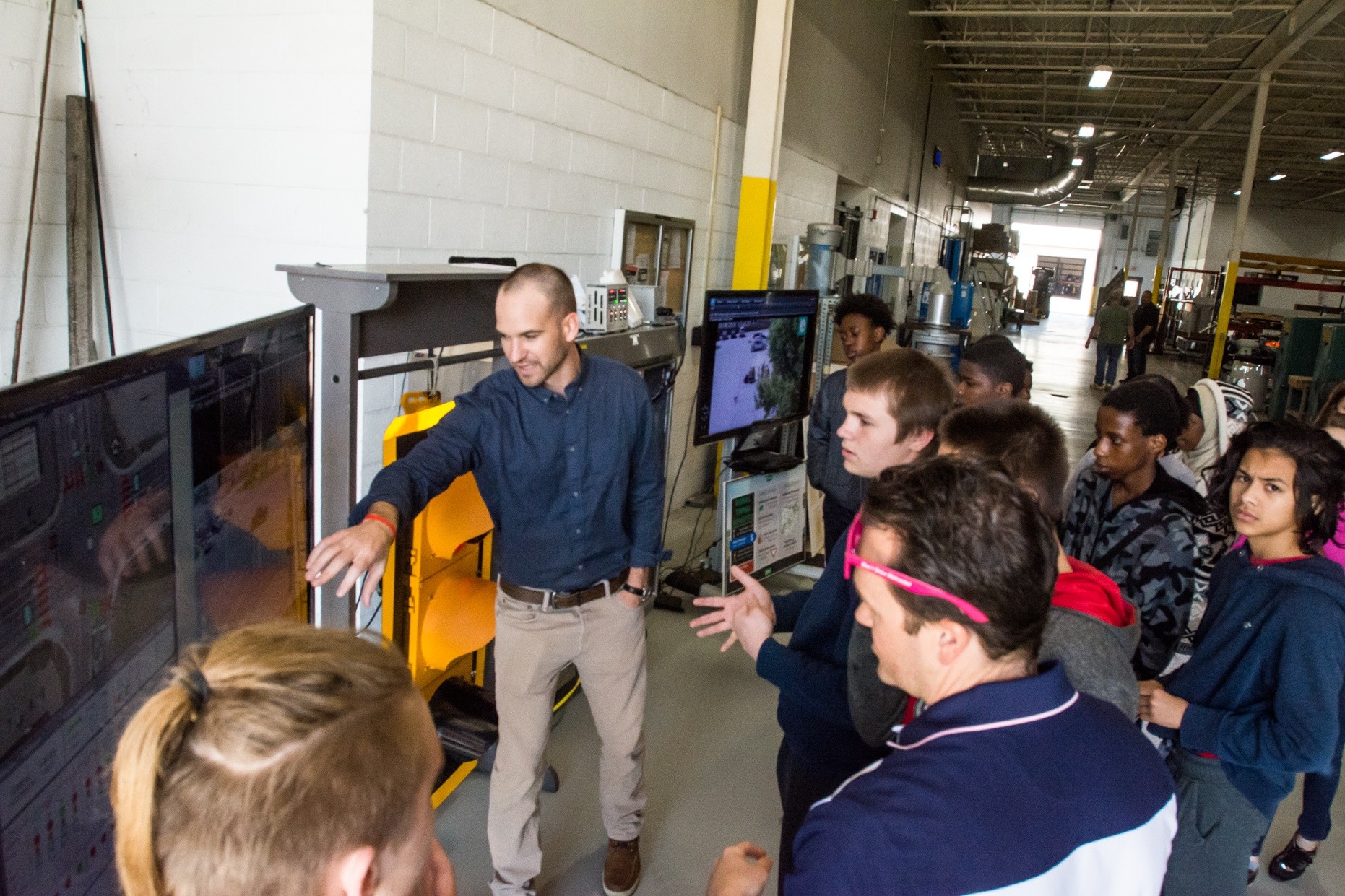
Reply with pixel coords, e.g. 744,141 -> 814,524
1105,384 -> 1111,391
1090,383 -> 1104,390
1119,378 -> 1128,384
1268,827 -> 1318,882
602,835 -> 640,896
1247,867 -> 1259,888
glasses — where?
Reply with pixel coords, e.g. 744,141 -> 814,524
843,504 -> 992,622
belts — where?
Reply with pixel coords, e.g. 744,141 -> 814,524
498,574 -> 626,610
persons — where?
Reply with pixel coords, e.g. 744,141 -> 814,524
305,262 -> 662,896
709,451 -> 1180,896
69,348 -> 299,588
1135,420 -> 1345,896
109,625 -> 455,896
686,288 -> 1345,884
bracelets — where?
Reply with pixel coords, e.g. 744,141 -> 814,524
362,513 -> 397,544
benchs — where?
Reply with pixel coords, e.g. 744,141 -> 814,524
1283,376 -> 1314,422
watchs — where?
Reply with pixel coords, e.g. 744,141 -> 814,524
622,582 -> 651,602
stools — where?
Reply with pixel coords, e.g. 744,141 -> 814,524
1001,308 -> 1024,330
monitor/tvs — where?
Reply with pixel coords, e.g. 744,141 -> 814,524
693,289 -> 820,446
722,459 -> 808,597
0,303 -> 318,896
